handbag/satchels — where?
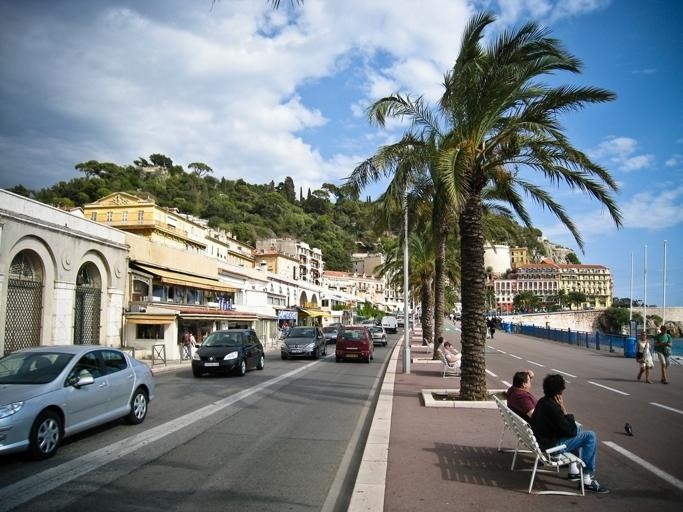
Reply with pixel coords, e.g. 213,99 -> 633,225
636,351 -> 645,364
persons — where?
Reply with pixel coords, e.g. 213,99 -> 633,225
636,332 -> 654,384
453,315 -> 456,326
507,370 -> 582,432
654,326 -> 673,384
486,318 -> 496,338
438,337 -> 462,368
189,334 -> 196,347
531,374 -> 610,494
183,332 -> 192,359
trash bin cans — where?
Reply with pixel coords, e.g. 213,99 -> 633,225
623,337 -> 638,358
504,322 -> 511,333
513,325 -> 517,334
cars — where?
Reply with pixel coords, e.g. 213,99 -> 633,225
280,326 -> 328,360
321,326 -> 337,343
191,329 -> 264,378
0,343 -> 154,456
369,326 -> 388,347
360,319 -> 381,326
329,323 -> 342,330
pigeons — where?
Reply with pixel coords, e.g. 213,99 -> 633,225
624,422 -> 632,436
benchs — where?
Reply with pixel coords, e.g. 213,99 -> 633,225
435,349 -> 459,377
424,338 -> 433,355
489,395 -> 587,497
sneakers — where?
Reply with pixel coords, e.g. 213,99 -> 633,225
568,472 -> 595,482
578,479 -> 610,495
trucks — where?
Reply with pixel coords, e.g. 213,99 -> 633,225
452,302 -> 461,319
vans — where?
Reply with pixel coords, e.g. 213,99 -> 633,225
381,316 -> 397,333
334,326 -> 374,362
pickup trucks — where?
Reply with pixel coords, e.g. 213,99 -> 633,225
396,314 -> 404,327
